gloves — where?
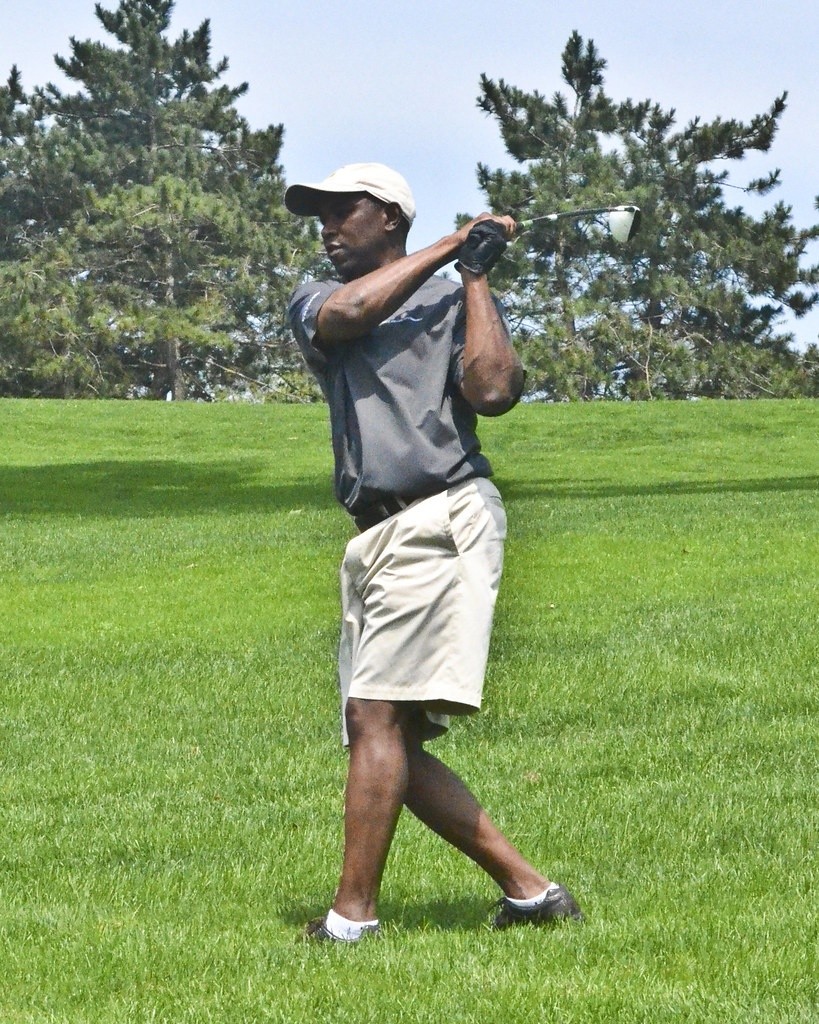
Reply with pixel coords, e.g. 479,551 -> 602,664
454,219 -> 507,277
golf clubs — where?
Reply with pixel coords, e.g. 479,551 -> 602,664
510,202 -> 643,245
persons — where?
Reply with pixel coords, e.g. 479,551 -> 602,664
285,164 -> 582,945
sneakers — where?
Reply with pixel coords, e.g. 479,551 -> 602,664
293,916 -> 385,944
487,884 -> 585,931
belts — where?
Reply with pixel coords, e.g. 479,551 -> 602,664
354,494 -> 423,533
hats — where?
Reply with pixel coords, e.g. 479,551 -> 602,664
284,162 -> 416,228
